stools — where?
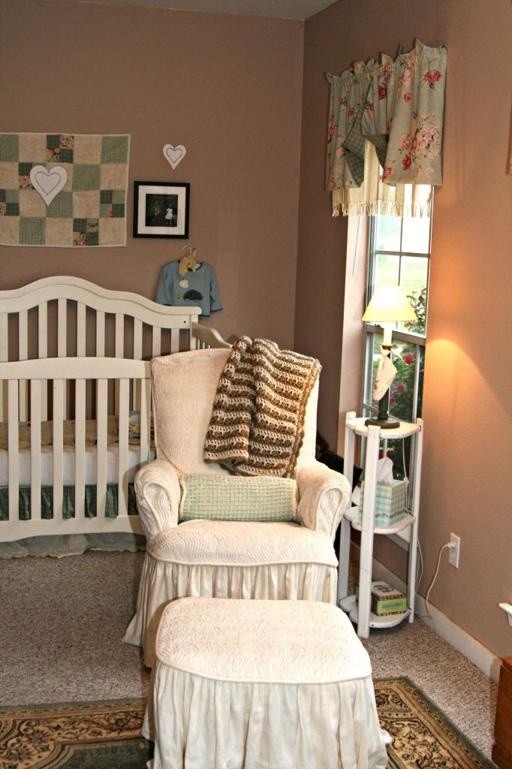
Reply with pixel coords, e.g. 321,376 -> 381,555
154,597 -> 383,768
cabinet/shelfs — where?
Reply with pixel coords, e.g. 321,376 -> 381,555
336,411 -> 424,639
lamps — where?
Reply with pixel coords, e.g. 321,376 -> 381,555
361,284 -> 420,430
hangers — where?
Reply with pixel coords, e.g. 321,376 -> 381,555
179,245 -> 201,267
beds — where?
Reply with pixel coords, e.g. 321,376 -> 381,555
1,274 -> 233,544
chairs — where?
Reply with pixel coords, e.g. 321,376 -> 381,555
134,345 -> 354,672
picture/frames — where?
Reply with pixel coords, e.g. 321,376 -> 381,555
132,180 -> 191,239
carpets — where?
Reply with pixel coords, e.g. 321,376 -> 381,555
1,676 -> 500,768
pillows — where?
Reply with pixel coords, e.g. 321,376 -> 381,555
177,474 -> 300,523
